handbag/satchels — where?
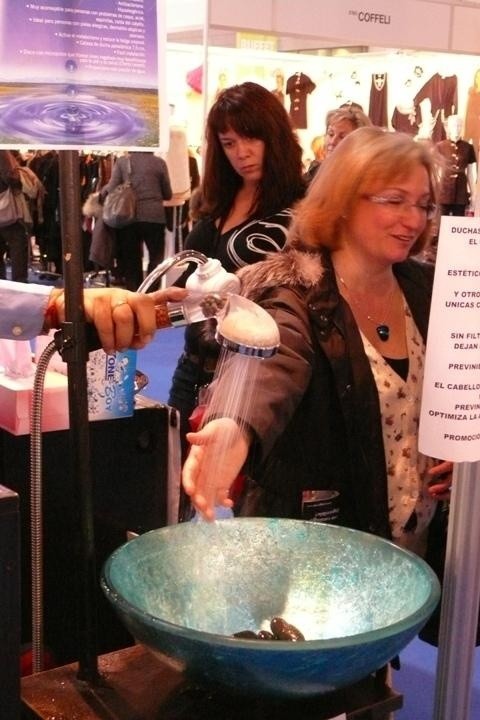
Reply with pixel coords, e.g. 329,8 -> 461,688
0,190 -> 17,226
103,184 -> 134,228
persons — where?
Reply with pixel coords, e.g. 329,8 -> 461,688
0,82 -> 480,720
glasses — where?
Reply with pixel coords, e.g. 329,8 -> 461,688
359,191 -> 439,221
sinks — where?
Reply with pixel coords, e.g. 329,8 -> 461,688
94,517 -> 442,701
